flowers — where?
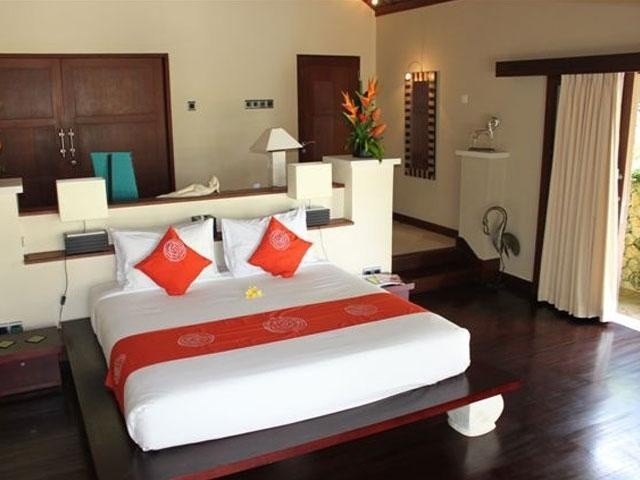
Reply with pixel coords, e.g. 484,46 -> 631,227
339,72 -> 392,164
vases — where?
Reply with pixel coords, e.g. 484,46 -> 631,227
353,144 -> 375,157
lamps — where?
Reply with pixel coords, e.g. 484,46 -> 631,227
249,127 -> 304,188
54,174 -> 111,256
405,61 -> 423,80
286,160 -> 334,231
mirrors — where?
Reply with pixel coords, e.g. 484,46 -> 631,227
403,71 -> 436,182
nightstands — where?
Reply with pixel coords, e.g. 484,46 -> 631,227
384,284 -> 415,301
1,326 -> 66,398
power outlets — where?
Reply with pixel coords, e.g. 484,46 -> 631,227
362,267 -> 372,275
373,266 -> 383,274
0,324 -> 8,335
9,321 -> 23,334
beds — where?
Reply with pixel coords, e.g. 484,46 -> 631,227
56,256 -> 523,480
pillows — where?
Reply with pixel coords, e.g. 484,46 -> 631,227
134,223 -> 214,298
245,214 -> 316,278
219,206 -> 317,279
105,215 -> 223,291
